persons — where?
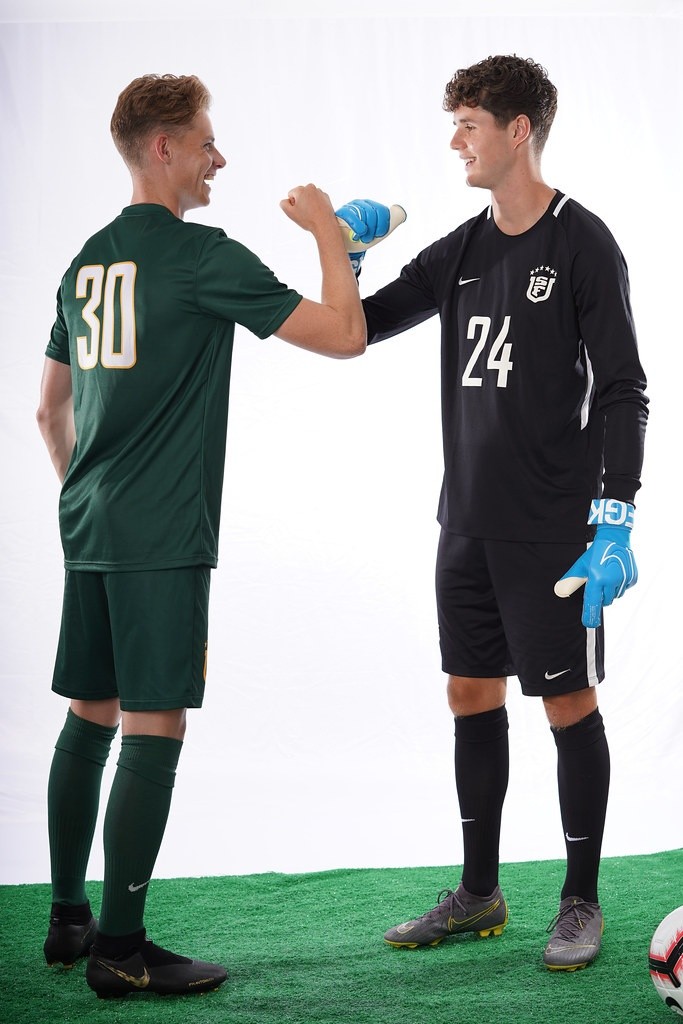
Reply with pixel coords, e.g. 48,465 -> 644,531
333,54 -> 649,973
34,68 -> 367,1004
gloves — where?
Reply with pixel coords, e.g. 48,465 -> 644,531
330,199 -> 405,280
556,499 -> 640,633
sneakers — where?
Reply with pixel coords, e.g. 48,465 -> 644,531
382,880 -> 510,949
43,915 -> 98,969
86,944 -> 230,1000
543,896 -> 605,970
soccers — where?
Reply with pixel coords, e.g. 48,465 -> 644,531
647,903 -> 683,1016
